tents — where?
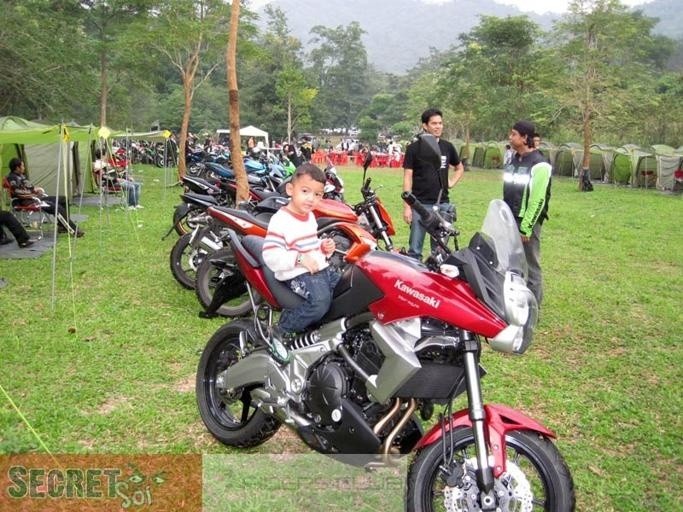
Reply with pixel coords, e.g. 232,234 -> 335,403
446,138 -> 683,196
0,116 -> 116,205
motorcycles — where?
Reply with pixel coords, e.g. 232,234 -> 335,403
194,132 -> 577,512
113,137 -> 399,318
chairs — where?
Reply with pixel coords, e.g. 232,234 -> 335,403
2,148 -> 130,238
311,149 -> 405,168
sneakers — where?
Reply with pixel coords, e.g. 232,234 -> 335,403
135,205 -> 143,208
1,238 -> 14,246
73,232 -> 84,238
266,322 -> 290,344
59,228 -> 67,232
19,240 -> 35,248
128,206 -> 134,210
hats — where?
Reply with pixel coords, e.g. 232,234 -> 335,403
514,122 -> 538,136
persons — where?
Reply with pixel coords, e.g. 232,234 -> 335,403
91,149 -> 144,212
532,133 -> 544,156
4,157 -> 84,238
260,163 -> 336,363
130,132 -> 401,168
501,121 -> 553,323
0,210 -> 35,248
400,108 -> 464,263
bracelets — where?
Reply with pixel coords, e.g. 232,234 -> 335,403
296,254 -> 303,264
